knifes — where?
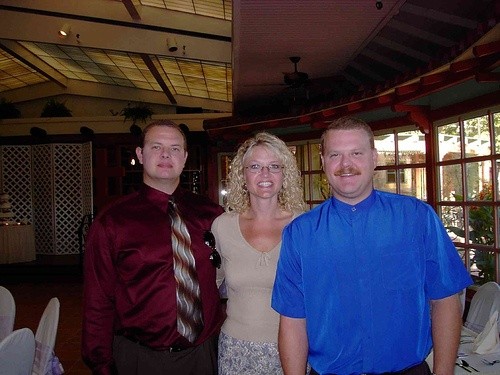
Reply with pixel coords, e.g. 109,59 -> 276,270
455,363 -> 472,373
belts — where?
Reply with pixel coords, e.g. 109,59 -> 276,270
169,343 -> 191,353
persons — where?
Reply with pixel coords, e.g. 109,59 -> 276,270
207,132 -> 311,375
80,120 -> 225,375
270,117 -> 474,375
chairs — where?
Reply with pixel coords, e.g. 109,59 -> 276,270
467,282 -> 500,334
0,286 -> 16,339
32,297 -> 60,375
0,328 -> 35,375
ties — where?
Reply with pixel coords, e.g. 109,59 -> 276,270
167,200 -> 206,345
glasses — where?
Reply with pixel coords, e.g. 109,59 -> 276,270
204,230 -> 221,271
243,160 -> 284,173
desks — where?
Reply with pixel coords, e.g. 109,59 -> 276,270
426,326 -> 500,375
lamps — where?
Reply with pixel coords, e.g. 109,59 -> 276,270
58,23 -> 72,36
167,35 -> 186,54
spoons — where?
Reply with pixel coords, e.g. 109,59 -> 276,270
461,360 -> 479,372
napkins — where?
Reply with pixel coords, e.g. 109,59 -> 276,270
473,311 -> 499,354
